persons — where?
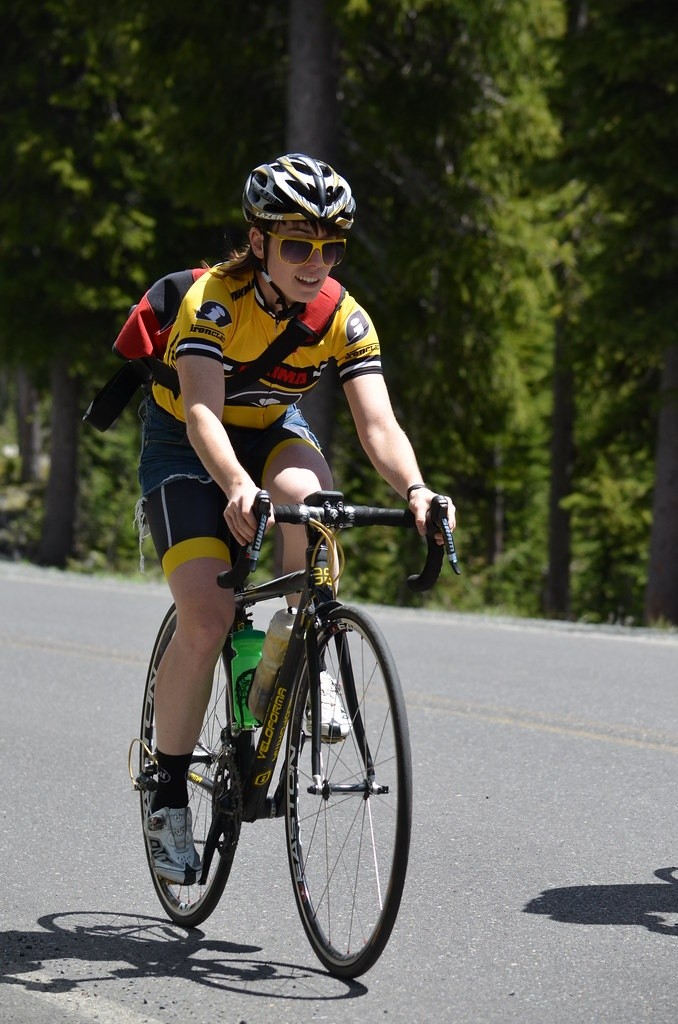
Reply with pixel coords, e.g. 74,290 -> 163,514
140,153 -> 456,885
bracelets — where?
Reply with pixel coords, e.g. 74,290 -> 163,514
407,485 -> 426,503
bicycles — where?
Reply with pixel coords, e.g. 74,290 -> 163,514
127,487 -> 463,980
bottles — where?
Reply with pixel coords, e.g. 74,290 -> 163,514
231,620 -> 267,726
248,606 -> 298,721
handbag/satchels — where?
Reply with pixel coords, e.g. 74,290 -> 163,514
82,261 -> 346,432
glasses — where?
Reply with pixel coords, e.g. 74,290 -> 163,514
261,229 -> 346,267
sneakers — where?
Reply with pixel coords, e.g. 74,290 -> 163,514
305,670 -> 353,737
144,791 -> 203,886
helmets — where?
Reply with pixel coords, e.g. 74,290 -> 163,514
241,154 -> 356,229
